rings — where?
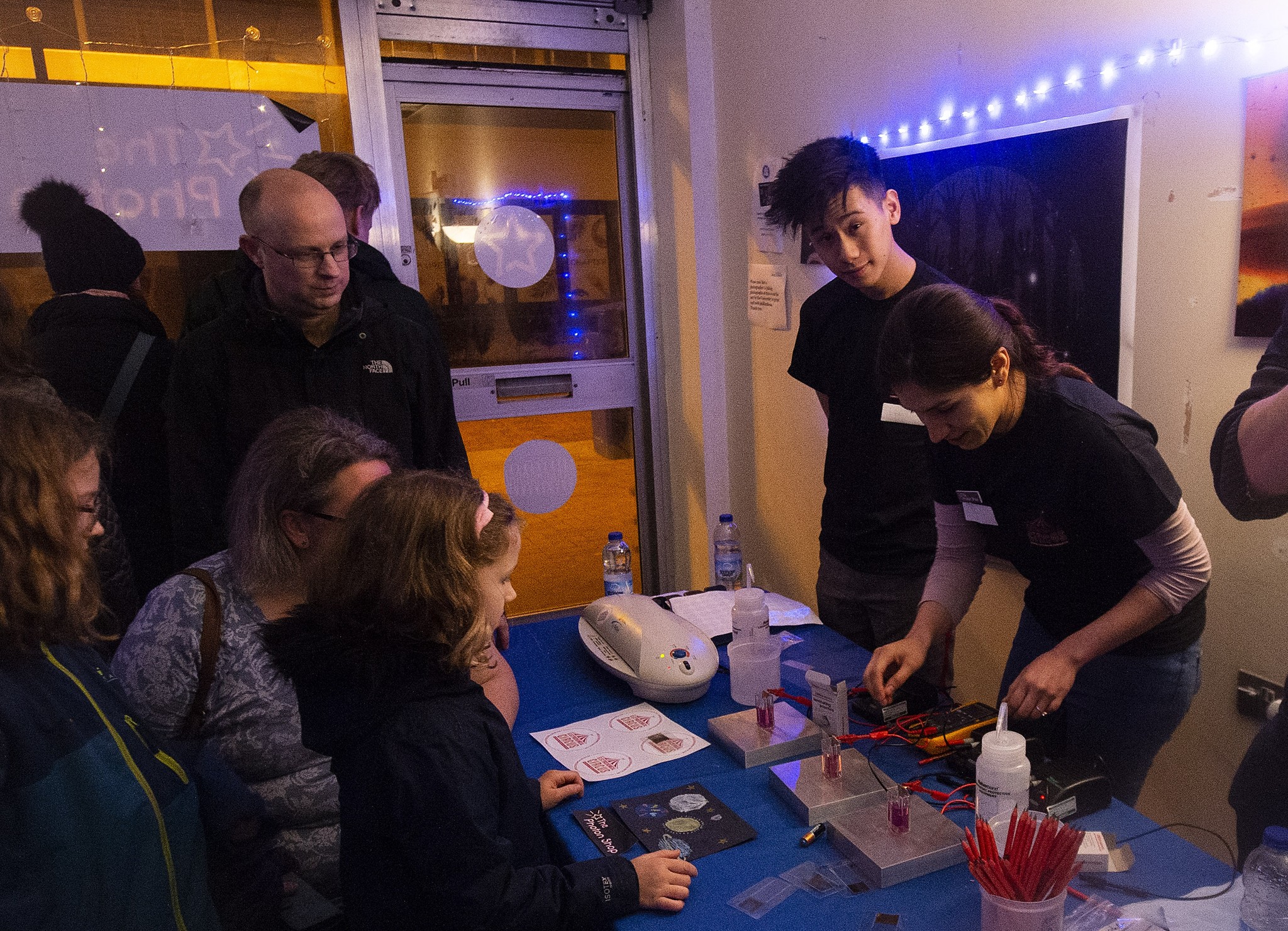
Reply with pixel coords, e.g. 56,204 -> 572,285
1036,705 -> 1047,716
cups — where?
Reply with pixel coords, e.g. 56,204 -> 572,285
978,883 -> 1067,931
988,808 -> 1065,859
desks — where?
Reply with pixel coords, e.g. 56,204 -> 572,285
494,591 -> 1238,931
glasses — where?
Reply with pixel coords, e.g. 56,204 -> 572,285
249,229 -> 360,269
58,495 -> 102,520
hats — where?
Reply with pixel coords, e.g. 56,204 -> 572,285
17,179 -> 146,296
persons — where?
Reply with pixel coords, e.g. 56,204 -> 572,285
253,469 -> 698,930
863,281 -> 1213,806
762,135 -> 954,691
1209,318 -> 1288,870
0,353 -> 218,930
153,168 -> 447,586
16,177 -> 172,651
113,407 -> 397,930
184,150 -> 472,477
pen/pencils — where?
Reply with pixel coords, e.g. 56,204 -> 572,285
959,802 -> 1086,931
1067,885 -> 1119,918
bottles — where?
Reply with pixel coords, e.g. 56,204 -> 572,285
727,637 -> 781,706
602,531 -> 633,596
713,514 -> 743,591
731,563 -> 770,640
974,703 -> 1030,853
1237,825 -> 1288,931
